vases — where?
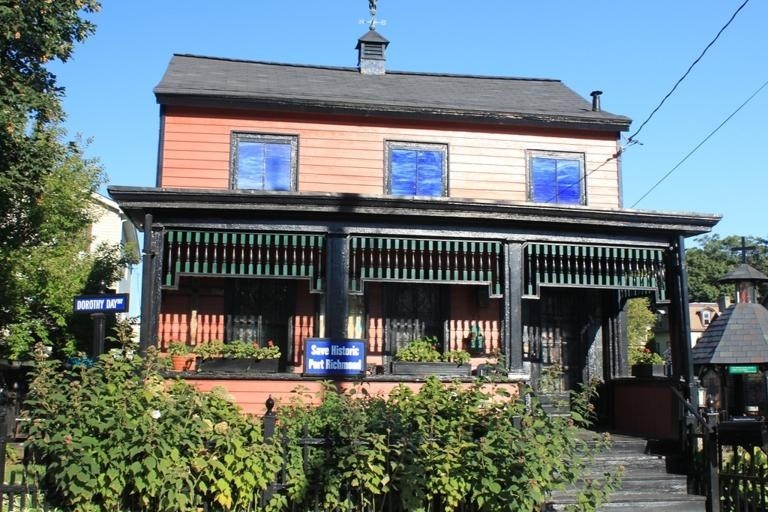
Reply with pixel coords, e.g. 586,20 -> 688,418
631,348 -> 663,364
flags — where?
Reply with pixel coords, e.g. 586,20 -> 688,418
632,365 -> 666,377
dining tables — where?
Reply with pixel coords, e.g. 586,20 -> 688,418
166,342 -> 193,372
392,335 -> 471,377
193,340 -> 281,373
